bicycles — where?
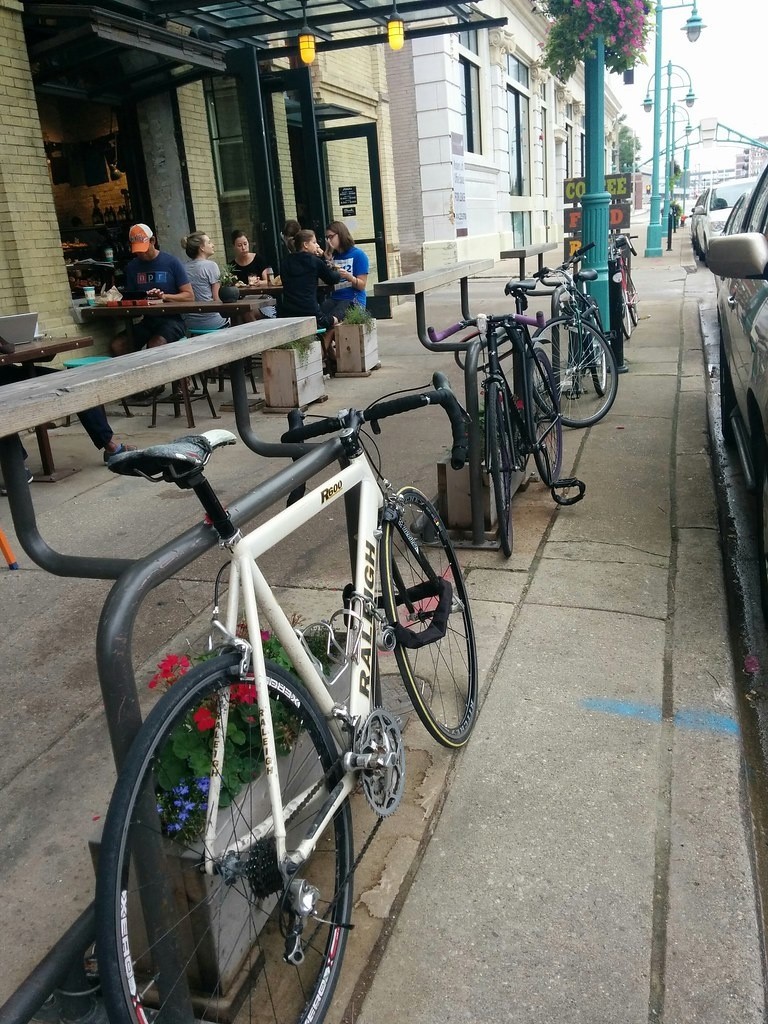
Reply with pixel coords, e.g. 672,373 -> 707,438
427,278 -> 585,559
87,369 -> 482,1024
605,233 -> 650,337
527,241 -> 629,429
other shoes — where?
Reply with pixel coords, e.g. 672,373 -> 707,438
134,383 -> 165,401
174,383 -> 195,401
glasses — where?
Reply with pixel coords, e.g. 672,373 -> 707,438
324,234 -> 336,241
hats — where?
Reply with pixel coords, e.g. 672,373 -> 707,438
129,223 -> 152,253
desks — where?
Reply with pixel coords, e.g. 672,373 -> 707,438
0,335 -> 93,482
89,299 -> 277,405
225,284 -> 353,369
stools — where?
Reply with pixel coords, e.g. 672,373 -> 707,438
63,356 -> 134,426
147,330 -> 260,430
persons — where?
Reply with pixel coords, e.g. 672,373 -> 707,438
0,364 -> 139,495
110,223 -> 194,402
179,220 -> 369,370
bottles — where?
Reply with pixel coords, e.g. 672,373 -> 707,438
71,263 -> 83,283
103,205 -> 133,222
110,238 -> 136,260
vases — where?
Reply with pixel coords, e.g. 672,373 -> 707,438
88,633 -> 367,998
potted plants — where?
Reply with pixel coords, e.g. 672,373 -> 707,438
333,303 -> 380,372
260,334 -> 326,409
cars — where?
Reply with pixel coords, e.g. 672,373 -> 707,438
684,161 -> 768,566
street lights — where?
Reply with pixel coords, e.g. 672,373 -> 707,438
658,102 -> 693,228
644,0 -> 707,258
639,61 -> 698,237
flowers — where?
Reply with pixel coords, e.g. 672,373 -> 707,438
537,0 -> 660,83
141,612 -> 338,844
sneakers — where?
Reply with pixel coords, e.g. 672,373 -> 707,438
1,468 -> 34,494
104,443 -> 138,465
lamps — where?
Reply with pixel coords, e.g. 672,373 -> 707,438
387,0 -> 405,50
297,0 -> 316,67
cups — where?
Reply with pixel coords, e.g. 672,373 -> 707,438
248,275 -> 258,287
266,273 -> 274,286
82,286 -> 95,306
